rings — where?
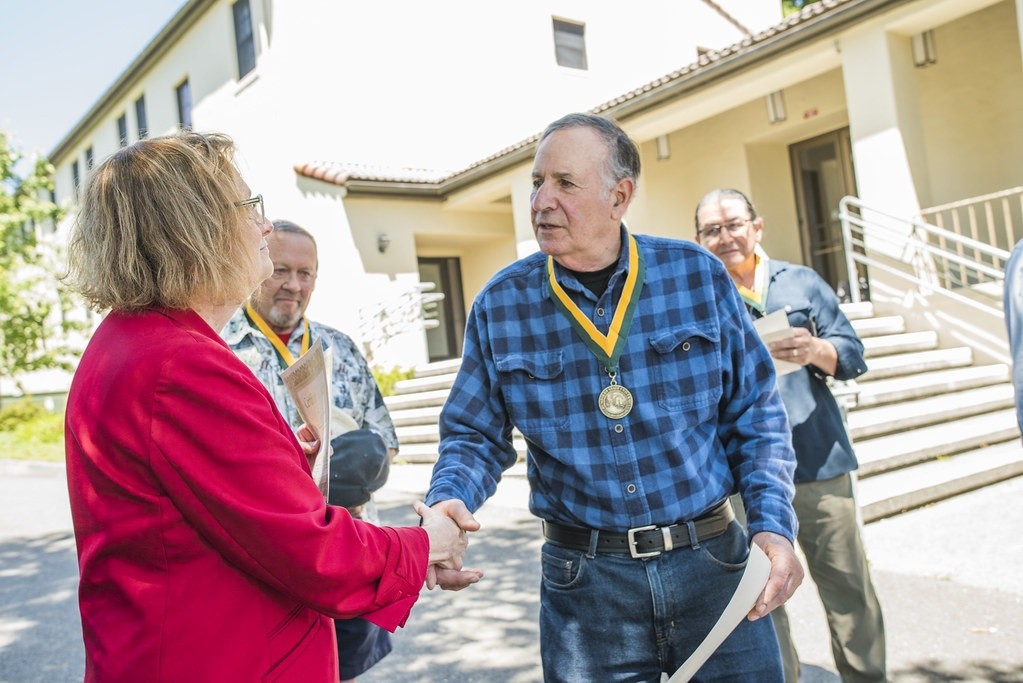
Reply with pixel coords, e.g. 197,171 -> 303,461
793,349 -> 797,356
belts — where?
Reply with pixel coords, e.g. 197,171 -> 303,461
544,498 -> 736,559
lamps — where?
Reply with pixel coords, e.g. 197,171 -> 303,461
910,28 -> 938,69
657,135 -> 670,162
378,233 -> 390,253
765,90 -> 787,125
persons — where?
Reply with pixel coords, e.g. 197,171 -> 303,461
694,188 -> 887,683
64,133 -> 467,683
420,111 -> 805,683
218,220 -> 399,683
1002,239 -> 1023,436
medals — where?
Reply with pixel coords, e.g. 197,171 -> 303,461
598,385 -> 633,419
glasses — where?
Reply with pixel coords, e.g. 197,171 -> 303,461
698,218 -> 752,238
225,194 -> 265,224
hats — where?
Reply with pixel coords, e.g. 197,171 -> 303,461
327,428 -> 390,505
